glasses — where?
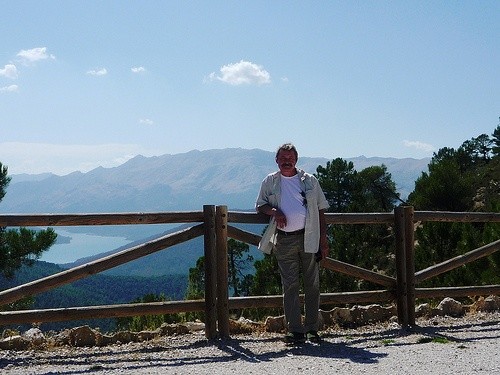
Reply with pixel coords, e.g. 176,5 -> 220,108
299,191 -> 307,206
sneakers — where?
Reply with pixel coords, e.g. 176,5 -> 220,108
307,330 -> 321,342
284,332 -> 306,342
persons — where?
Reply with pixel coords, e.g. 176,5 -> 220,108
255,143 -> 330,342
